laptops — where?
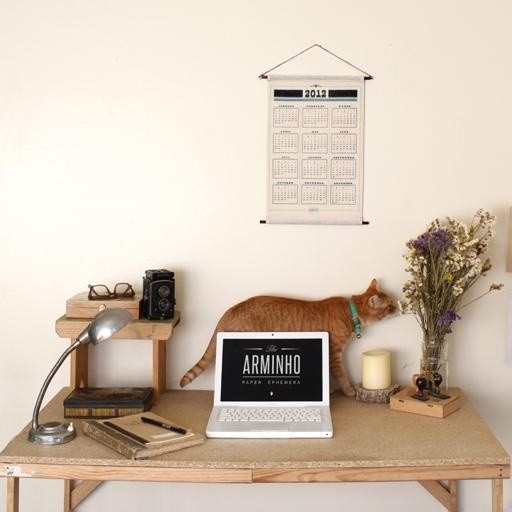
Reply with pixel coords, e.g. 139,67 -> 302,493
205,331 -> 333,439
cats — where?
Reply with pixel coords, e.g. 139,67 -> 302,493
178,280 -> 403,397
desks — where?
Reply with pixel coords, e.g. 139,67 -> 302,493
0,386 -> 511,512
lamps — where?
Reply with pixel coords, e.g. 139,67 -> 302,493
27,306 -> 134,446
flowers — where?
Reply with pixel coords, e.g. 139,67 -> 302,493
393,206 -> 505,366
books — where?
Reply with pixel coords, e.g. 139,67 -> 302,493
63,395 -> 152,417
64,385 -> 153,408
82,411 -> 206,459
102,411 -> 195,444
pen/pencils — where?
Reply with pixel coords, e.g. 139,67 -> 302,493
141,417 -> 187,434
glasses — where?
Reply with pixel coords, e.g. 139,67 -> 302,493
88,283 -> 135,300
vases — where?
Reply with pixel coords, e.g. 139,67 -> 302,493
419,339 -> 449,398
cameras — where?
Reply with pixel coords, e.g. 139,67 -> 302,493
142,269 -> 175,320
